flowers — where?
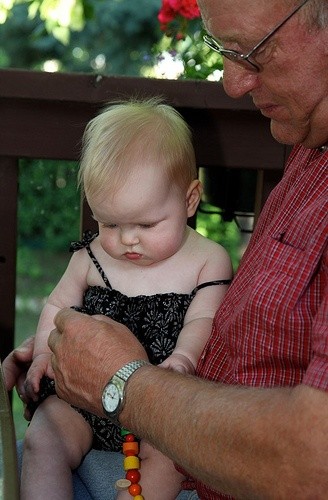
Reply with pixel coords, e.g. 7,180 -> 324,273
158,0 -> 219,80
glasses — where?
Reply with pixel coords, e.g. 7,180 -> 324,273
203,0 -> 308,73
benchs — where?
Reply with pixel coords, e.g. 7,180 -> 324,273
0,69 -> 294,409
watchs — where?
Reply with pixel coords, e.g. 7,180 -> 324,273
101,359 -> 152,427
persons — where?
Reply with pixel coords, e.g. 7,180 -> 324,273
1,0 -> 328,500
20,96 -> 234,500
